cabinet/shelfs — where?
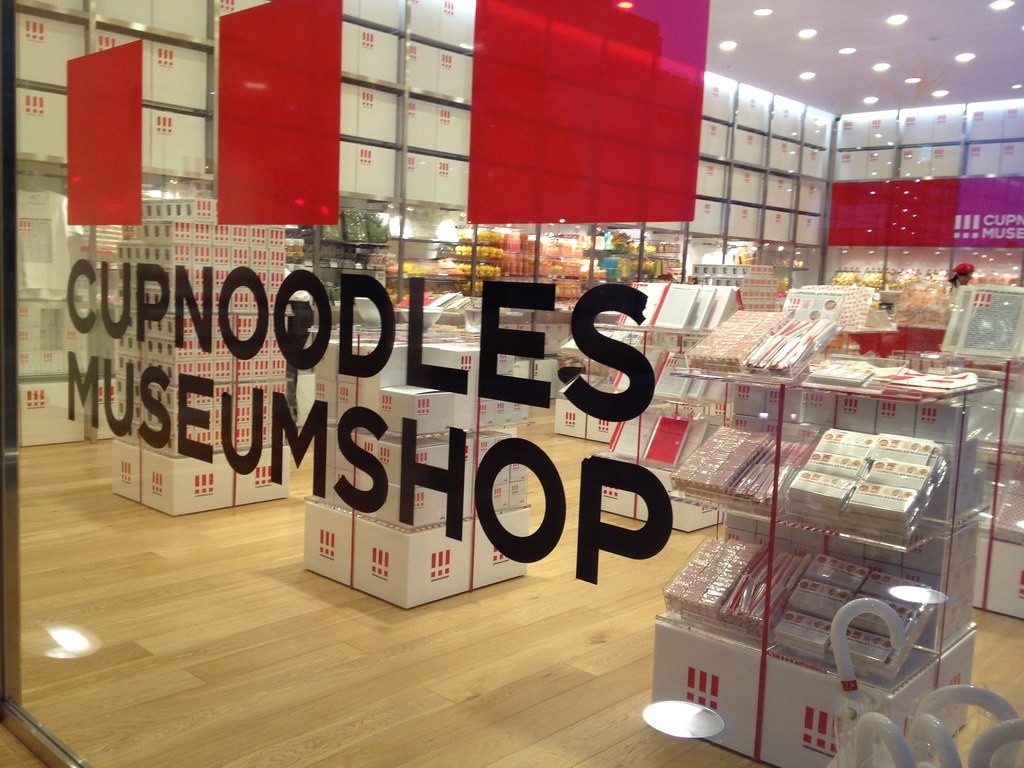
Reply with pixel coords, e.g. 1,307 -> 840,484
312,309 -> 618,398
655,369 -> 1002,691
15,0 -> 831,258
834,137 -> 1024,182
17,174 -> 119,442
945,352 -> 1024,547
303,417 -> 535,535
589,324 -> 733,472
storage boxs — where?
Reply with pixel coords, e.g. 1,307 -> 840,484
17,190 -> 1024,768
12,0 -> 832,246
834,102 -> 1024,182
